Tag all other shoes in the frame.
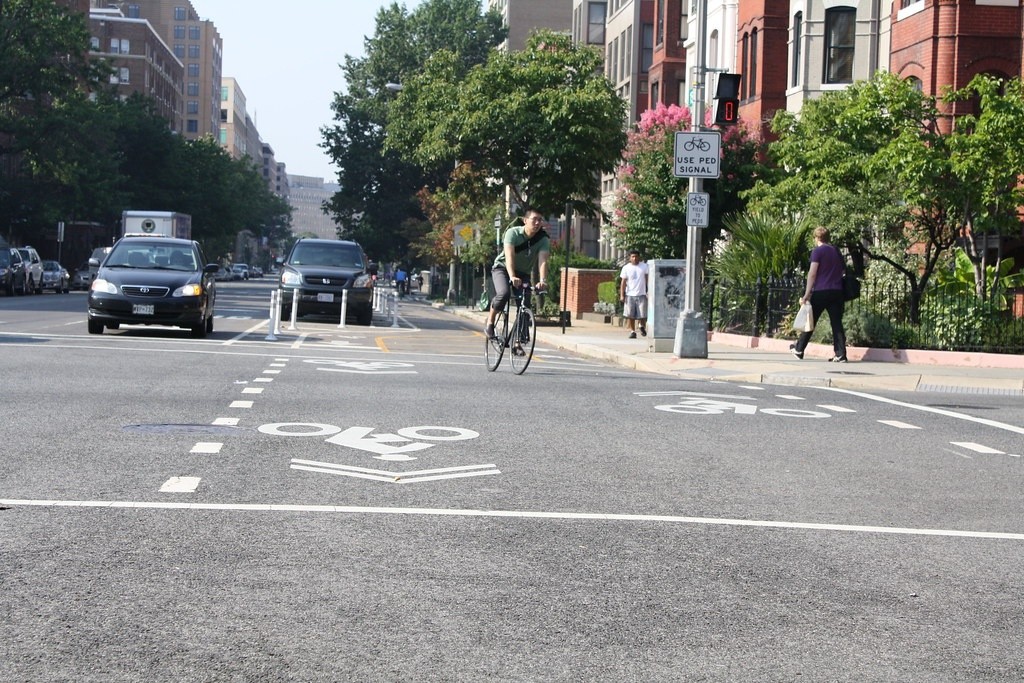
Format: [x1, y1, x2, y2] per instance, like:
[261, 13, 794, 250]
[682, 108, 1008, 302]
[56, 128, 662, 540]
[629, 332, 637, 339]
[512, 346, 526, 356]
[640, 327, 647, 337]
[484, 318, 495, 338]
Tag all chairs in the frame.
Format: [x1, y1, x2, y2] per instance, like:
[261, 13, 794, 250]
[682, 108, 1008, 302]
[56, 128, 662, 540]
[302, 249, 356, 269]
[155, 256, 169, 263]
[129, 252, 144, 266]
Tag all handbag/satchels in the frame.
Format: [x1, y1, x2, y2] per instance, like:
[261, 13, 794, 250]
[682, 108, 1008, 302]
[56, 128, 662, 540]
[501, 217, 549, 253]
[841, 274, 862, 301]
[792, 298, 814, 332]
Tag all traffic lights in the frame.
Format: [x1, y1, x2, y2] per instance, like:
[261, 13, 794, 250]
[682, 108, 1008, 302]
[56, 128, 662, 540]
[714, 72, 742, 125]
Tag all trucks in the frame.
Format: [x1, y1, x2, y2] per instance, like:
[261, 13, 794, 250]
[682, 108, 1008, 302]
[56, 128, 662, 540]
[118, 209, 192, 268]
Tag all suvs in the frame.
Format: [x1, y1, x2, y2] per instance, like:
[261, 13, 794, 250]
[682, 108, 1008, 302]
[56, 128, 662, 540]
[14, 245, 44, 296]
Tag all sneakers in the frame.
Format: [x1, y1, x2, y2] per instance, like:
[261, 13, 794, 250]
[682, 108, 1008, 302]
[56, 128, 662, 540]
[790, 344, 804, 360]
[829, 355, 847, 363]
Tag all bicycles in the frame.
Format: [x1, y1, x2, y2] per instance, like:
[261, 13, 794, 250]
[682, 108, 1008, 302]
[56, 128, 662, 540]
[483, 278, 548, 376]
[395, 280, 406, 301]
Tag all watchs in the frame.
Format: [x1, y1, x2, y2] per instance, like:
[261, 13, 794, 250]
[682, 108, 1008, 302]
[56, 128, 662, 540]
[539, 278, 547, 283]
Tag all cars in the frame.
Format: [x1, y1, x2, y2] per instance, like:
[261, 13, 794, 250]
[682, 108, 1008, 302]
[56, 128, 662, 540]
[0, 242, 28, 298]
[71, 261, 90, 291]
[41, 259, 70, 294]
[273, 235, 376, 327]
[86, 232, 220, 338]
[210, 262, 264, 282]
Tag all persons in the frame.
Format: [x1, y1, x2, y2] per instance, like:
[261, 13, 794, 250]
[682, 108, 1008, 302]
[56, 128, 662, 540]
[397, 269, 407, 297]
[417, 273, 423, 293]
[620, 251, 649, 338]
[483, 208, 549, 357]
[789, 226, 848, 362]
